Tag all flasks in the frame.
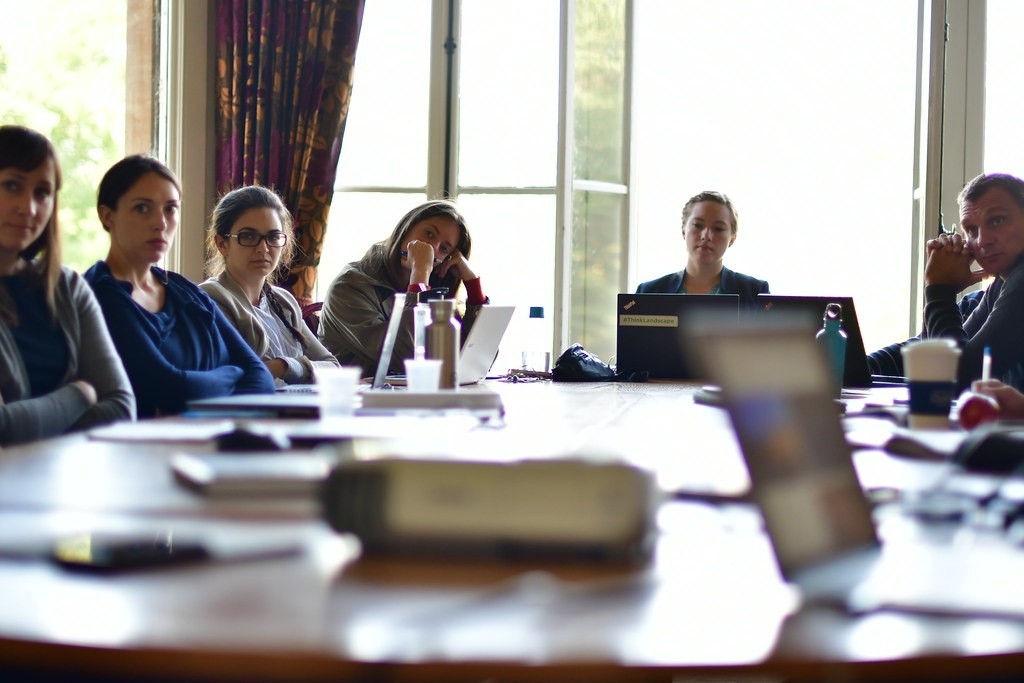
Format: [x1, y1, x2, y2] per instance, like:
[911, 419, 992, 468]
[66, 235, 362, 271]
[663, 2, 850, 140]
[423, 297, 457, 392]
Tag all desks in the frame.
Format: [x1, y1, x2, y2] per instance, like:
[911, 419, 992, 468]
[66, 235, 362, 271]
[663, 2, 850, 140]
[0, 381, 1024, 683]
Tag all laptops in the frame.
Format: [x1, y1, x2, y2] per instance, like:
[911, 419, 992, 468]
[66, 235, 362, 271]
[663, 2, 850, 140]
[618, 294, 1024, 625]
[364, 305, 515, 385]
[273, 294, 406, 396]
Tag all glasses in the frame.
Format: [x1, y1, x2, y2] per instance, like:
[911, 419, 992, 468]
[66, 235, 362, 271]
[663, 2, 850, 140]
[223, 231, 288, 248]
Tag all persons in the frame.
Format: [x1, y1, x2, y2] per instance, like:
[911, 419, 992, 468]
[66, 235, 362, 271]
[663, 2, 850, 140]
[195, 186, 340, 385]
[867, 173, 1024, 423]
[76, 154, 275, 419]
[636, 191, 771, 295]
[0, 124, 136, 447]
[319, 200, 489, 375]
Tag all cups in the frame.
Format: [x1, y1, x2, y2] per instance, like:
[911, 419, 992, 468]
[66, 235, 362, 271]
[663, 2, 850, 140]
[403, 360, 444, 392]
[311, 366, 364, 420]
[899, 336, 962, 432]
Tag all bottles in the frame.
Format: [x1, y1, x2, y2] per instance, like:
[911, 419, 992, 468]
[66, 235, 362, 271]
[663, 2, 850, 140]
[815, 303, 847, 396]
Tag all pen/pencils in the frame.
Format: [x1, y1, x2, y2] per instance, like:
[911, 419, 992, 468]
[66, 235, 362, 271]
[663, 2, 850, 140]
[982, 347, 991, 380]
[401, 251, 443, 262]
